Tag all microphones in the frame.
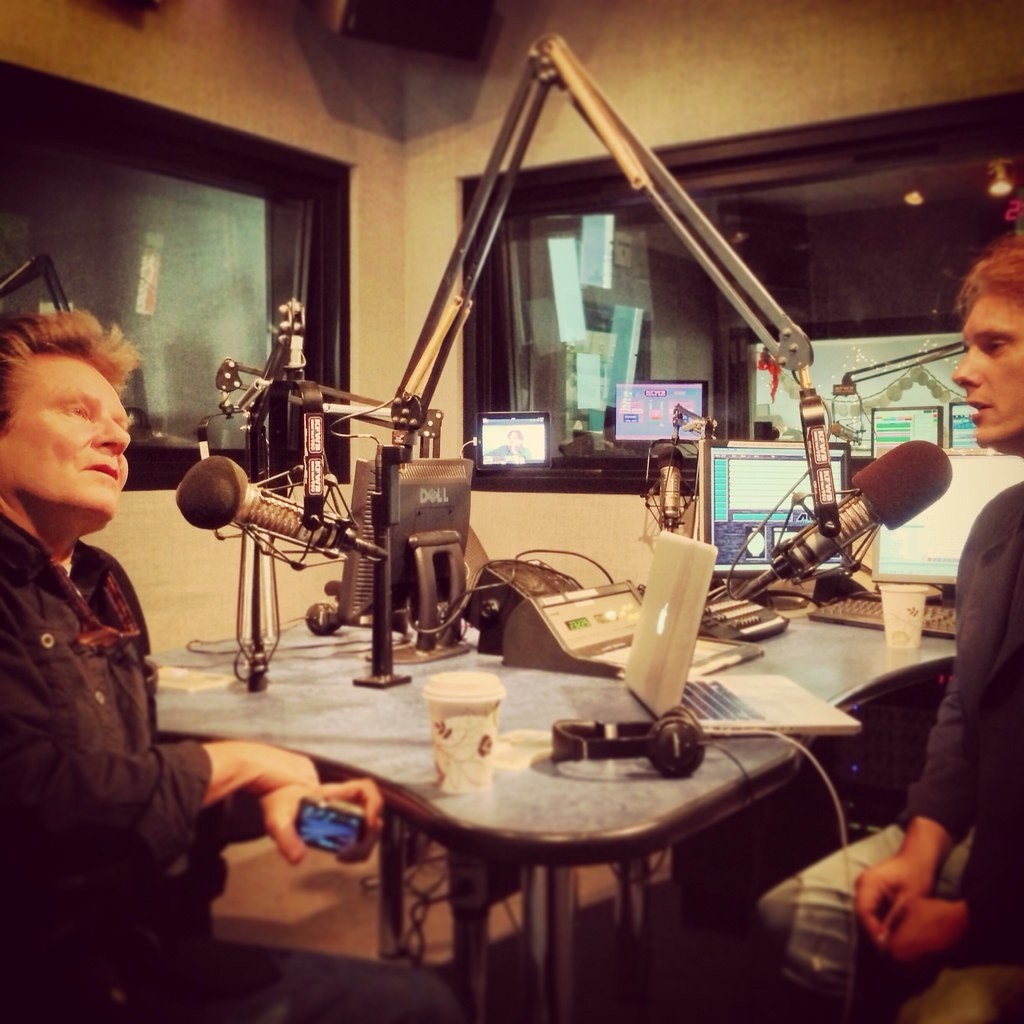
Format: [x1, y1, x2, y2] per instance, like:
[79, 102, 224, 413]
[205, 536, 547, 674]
[175, 455, 389, 560]
[736, 441, 953, 601]
[655, 445, 685, 529]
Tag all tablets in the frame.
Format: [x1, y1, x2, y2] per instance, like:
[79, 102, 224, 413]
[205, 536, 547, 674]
[476, 411, 552, 470]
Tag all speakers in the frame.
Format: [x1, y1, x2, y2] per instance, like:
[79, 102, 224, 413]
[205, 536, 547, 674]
[320, 0, 494, 62]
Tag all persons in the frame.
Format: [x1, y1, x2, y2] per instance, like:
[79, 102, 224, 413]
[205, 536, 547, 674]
[496, 433, 533, 465]
[0, 309, 386, 1024]
[755, 233, 1024, 1024]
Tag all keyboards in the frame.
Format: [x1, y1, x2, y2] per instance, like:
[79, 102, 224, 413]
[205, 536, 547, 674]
[807, 599, 959, 640]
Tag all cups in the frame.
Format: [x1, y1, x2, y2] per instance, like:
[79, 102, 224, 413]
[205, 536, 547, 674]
[423, 670, 506, 795]
[879, 584, 929, 650]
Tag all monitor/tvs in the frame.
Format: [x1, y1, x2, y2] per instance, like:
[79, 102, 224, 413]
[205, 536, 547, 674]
[611, 380, 709, 446]
[949, 401, 982, 450]
[336, 456, 475, 662]
[871, 405, 942, 459]
[872, 448, 1024, 607]
[698, 440, 853, 609]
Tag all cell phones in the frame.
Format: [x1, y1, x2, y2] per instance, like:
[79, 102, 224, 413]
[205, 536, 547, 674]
[297, 793, 366, 854]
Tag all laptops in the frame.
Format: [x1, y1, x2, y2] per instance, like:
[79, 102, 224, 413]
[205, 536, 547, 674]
[626, 532, 863, 736]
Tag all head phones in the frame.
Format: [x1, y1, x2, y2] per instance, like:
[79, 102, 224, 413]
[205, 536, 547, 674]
[550, 704, 707, 777]
[304, 602, 377, 635]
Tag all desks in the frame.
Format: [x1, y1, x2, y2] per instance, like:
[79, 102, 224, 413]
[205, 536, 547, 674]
[150, 599, 958, 1024]
